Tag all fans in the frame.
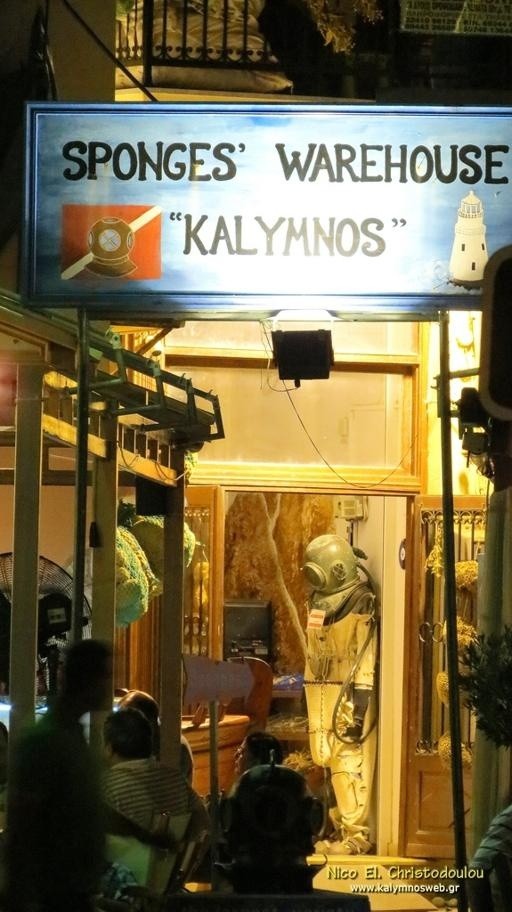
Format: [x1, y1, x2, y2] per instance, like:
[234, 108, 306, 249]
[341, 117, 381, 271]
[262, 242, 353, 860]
[0, 552, 92, 709]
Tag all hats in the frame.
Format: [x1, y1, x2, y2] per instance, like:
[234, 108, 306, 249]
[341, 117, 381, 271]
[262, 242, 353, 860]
[39, 595, 88, 640]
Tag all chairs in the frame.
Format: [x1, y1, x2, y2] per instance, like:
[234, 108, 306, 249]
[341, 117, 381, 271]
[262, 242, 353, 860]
[146, 789, 226, 896]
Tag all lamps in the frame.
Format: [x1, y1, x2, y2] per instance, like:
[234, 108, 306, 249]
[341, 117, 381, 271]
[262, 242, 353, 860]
[271, 328, 334, 387]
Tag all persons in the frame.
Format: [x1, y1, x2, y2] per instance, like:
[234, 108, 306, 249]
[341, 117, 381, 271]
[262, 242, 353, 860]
[301, 532, 379, 854]
[1, 638, 284, 912]
[467, 805, 512, 912]
